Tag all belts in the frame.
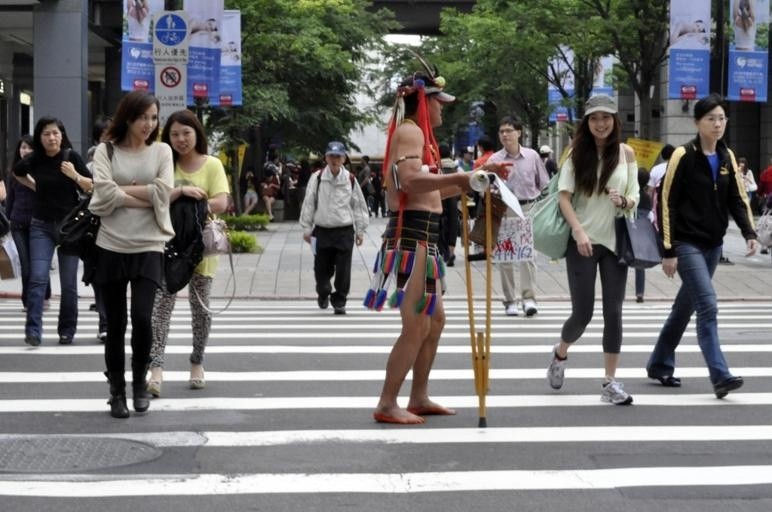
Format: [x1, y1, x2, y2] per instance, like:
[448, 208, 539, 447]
[519, 200, 534, 204]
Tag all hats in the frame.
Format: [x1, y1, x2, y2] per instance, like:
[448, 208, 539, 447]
[425, 87, 456, 102]
[325, 142, 346, 156]
[584, 94, 618, 116]
[540, 145, 554, 154]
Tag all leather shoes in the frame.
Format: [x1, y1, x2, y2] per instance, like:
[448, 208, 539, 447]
[189, 367, 206, 388]
[713, 376, 743, 399]
[147, 379, 161, 397]
[648, 375, 680, 387]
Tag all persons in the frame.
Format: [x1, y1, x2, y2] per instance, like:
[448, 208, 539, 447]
[758, 167, 772, 254]
[241, 171, 263, 216]
[733, 0, 757, 51]
[264, 152, 326, 215]
[190, 18, 217, 33]
[364, 76, 515, 423]
[299, 141, 369, 314]
[540, 145, 558, 179]
[720, 247, 733, 265]
[485, 115, 550, 317]
[261, 169, 280, 221]
[678, 20, 706, 37]
[438, 135, 495, 267]
[127, 0, 151, 45]
[89, 90, 174, 419]
[635, 168, 655, 302]
[737, 158, 755, 200]
[1, 115, 111, 346]
[548, 94, 640, 405]
[353, 156, 388, 218]
[648, 144, 675, 197]
[147, 111, 231, 399]
[645, 97, 758, 398]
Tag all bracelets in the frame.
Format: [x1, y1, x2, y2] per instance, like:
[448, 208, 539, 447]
[180, 185, 183, 195]
[75, 174, 79, 181]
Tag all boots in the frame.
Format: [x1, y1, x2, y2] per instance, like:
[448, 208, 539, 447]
[105, 371, 129, 418]
[131, 355, 153, 412]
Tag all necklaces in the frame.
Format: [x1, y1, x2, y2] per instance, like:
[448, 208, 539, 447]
[403, 120, 435, 155]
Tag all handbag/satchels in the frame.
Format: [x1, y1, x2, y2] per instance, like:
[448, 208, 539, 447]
[755, 216, 771, 248]
[202, 214, 228, 257]
[528, 173, 576, 260]
[615, 215, 661, 270]
[58, 198, 101, 263]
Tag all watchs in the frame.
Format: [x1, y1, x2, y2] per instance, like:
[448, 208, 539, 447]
[620, 196, 627, 209]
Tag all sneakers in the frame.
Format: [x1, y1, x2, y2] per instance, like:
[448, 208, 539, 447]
[600, 382, 632, 404]
[58, 335, 72, 344]
[97, 332, 107, 342]
[25, 333, 39, 346]
[506, 302, 518, 315]
[318, 296, 328, 308]
[334, 305, 345, 315]
[523, 298, 536, 316]
[547, 344, 567, 389]
